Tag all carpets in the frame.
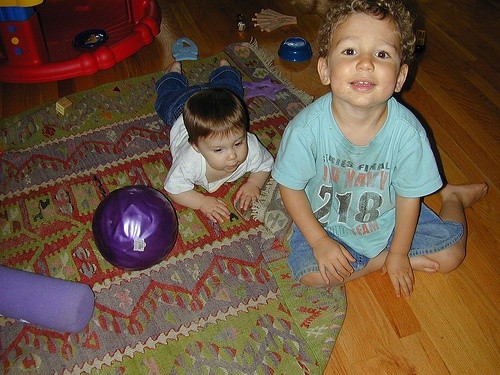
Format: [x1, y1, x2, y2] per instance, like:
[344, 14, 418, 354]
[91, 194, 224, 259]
[0, 37, 348, 375]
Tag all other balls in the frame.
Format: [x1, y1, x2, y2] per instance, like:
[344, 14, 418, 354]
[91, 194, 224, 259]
[91, 185, 179, 270]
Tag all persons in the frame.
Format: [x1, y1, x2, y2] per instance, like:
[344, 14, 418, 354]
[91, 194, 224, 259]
[271, 0, 488, 300]
[154, 58, 275, 223]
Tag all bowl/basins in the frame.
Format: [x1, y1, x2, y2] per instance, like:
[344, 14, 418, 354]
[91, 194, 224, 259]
[278, 37, 313, 62]
